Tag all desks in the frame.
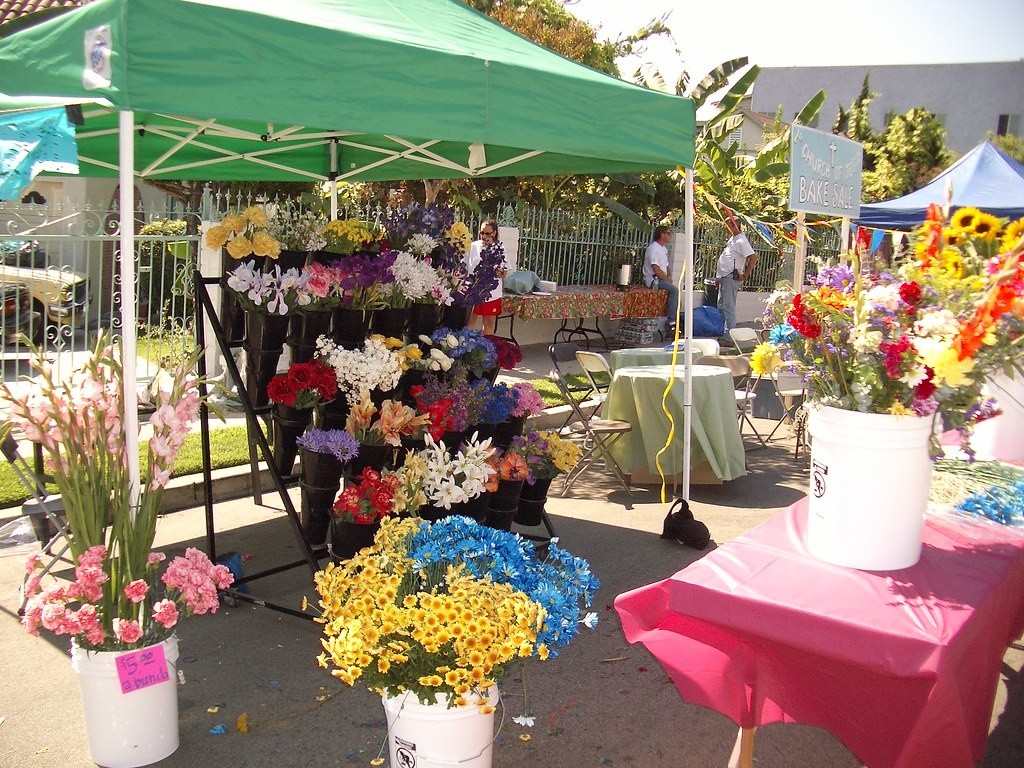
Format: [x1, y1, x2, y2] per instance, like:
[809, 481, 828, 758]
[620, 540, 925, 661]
[495, 284, 667, 351]
[597, 365, 748, 486]
[609, 347, 704, 370]
[615, 492, 1024, 768]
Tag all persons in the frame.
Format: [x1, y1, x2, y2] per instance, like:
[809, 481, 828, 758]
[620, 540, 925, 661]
[461, 219, 512, 335]
[716, 216, 756, 347]
[643, 225, 683, 340]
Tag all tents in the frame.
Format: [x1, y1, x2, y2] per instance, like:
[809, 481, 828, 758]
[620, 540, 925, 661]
[0, 0, 695, 530]
[851, 141, 1024, 235]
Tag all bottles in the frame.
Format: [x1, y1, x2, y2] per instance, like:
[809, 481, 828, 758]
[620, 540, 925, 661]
[653, 277, 658, 290]
[614, 317, 658, 345]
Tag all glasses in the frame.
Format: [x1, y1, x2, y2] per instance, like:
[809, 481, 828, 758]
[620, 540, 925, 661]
[664, 232, 671, 235]
[480, 230, 495, 236]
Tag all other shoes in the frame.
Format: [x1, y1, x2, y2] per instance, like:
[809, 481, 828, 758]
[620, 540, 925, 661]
[717, 337, 734, 347]
[666, 322, 681, 335]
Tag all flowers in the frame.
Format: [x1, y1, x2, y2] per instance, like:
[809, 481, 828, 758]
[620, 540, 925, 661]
[0, 327, 236, 652]
[205, 202, 600, 768]
[754, 197, 1024, 459]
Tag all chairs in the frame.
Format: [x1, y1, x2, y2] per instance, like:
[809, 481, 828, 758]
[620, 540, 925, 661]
[548, 343, 609, 437]
[729, 328, 773, 409]
[695, 354, 768, 452]
[574, 351, 616, 452]
[550, 370, 636, 498]
[766, 364, 808, 452]
[21, 435, 66, 554]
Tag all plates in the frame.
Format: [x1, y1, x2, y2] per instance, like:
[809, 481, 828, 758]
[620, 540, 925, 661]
[532, 292, 552, 296]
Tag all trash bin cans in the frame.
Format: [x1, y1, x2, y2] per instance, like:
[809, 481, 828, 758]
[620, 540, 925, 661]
[750, 370, 786, 420]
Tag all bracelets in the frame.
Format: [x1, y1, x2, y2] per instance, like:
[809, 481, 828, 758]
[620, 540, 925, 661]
[741, 273, 748, 280]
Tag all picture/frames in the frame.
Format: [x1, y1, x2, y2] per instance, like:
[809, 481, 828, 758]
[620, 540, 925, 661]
[381, 682, 500, 768]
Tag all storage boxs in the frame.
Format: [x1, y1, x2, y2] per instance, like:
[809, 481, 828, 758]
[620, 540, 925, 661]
[615, 314, 660, 345]
[749, 372, 805, 418]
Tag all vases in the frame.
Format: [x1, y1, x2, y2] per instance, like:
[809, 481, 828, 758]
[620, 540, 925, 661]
[408, 303, 441, 359]
[456, 491, 492, 526]
[370, 307, 409, 342]
[395, 439, 425, 468]
[419, 499, 457, 524]
[332, 518, 382, 567]
[805, 400, 940, 570]
[440, 302, 474, 332]
[515, 478, 551, 526]
[298, 447, 343, 547]
[488, 478, 525, 534]
[369, 387, 388, 426]
[332, 309, 372, 351]
[402, 370, 434, 409]
[287, 307, 333, 364]
[440, 431, 467, 457]
[240, 304, 291, 406]
[265, 249, 309, 278]
[344, 444, 394, 491]
[312, 252, 347, 266]
[316, 386, 351, 431]
[491, 415, 529, 454]
[467, 423, 495, 445]
[272, 402, 313, 476]
[468, 364, 501, 390]
[69, 636, 182, 768]
[221, 245, 268, 345]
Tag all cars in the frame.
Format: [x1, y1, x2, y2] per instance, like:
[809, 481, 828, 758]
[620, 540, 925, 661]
[0, 280, 41, 347]
[0, 264, 92, 345]
